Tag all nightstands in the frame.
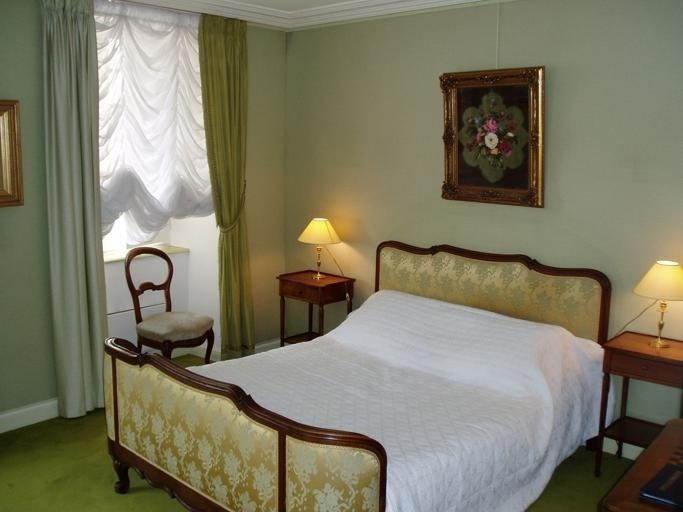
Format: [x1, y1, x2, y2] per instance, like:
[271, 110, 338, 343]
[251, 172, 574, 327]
[275, 269, 357, 347]
[594, 329, 683, 478]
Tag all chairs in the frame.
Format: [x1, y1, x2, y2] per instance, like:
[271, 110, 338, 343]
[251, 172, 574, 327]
[124, 246, 214, 364]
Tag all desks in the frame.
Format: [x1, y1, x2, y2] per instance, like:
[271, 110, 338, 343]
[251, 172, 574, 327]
[600, 418, 683, 512]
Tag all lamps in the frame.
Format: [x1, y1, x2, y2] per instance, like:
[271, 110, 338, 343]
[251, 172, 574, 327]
[297, 217, 342, 280]
[632, 258, 683, 348]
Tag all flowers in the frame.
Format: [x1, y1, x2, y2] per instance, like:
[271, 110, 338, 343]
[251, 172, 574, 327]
[458, 92, 527, 184]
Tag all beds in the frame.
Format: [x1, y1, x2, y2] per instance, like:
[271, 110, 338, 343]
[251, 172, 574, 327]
[101, 239, 616, 512]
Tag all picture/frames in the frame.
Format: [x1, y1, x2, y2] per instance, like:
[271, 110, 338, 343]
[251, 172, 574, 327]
[438, 64, 545, 208]
[0, 98, 24, 206]
